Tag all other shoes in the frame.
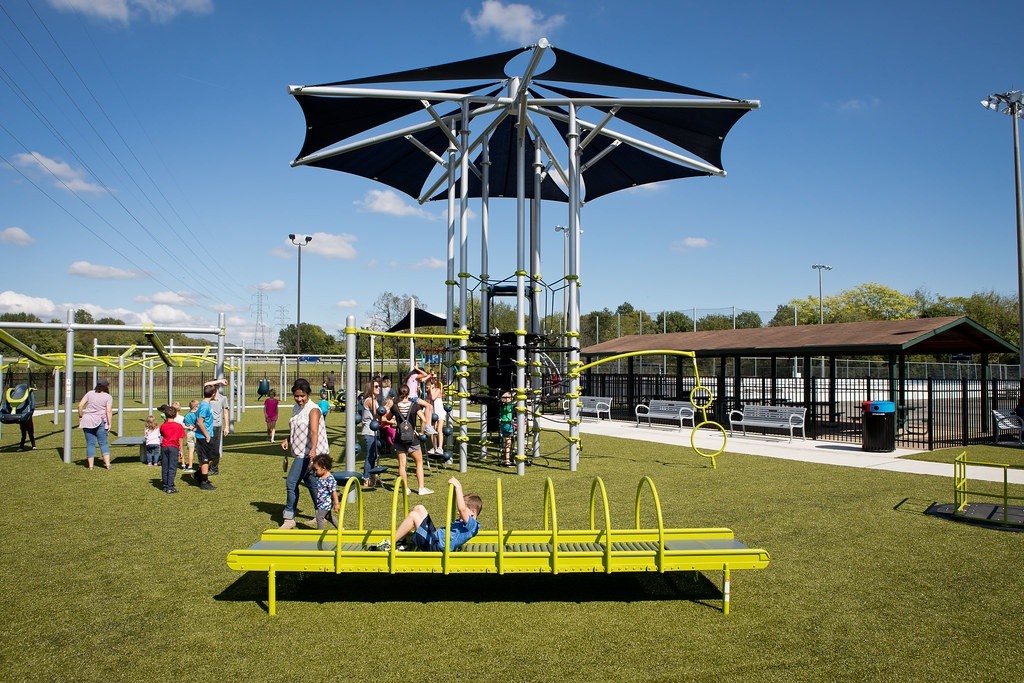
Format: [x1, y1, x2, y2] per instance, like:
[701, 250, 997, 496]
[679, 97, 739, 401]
[167, 489, 176, 493]
[148, 462, 152, 466]
[32, 447, 37, 450]
[369, 538, 393, 551]
[201, 481, 216, 489]
[187, 467, 193, 472]
[153, 463, 159, 466]
[406, 487, 411, 495]
[163, 486, 168, 491]
[425, 425, 438, 434]
[428, 448, 435, 454]
[181, 465, 186, 471]
[17, 448, 23, 452]
[270, 439, 275, 444]
[396, 538, 408, 551]
[89, 467, 94, 470]
[278, 519, 297, 530]
[419, 487, 433, 495]
[105, 464, 111, 469]
[435, 448, 443, 455]
[363, 479, 377, 486]
[211, 466, 218, 475]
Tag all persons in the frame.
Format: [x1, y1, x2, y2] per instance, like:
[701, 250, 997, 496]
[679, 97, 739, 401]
[322, 380, 327, 390]
[182, 400, 199, 472]
[499, 392, 517, 467]
[318, 393, 329, 420]
[387, 385, 435, 496]
[402, 364, 438, 435]
[16, 391, 36, 452]
[144, 416, 161, 465]
[381, 409, 396, 446]
[370, 477, 483, 551]
[380, 376, 399, 454]
[327, 370, 339, 400]
[312, 454, 345, 529]
[195, 385, 219, 491]
[361, 380, 391, 487]
[5, 384, 37, 414]
[204, 379, 230, 476]
[277, 379, 329, 530]
[160, 406, 186, 493]
[172, 400, 186, 470]
[263, 389, 279, 443]
[418, 378, 446, 455]
[77, 377, 113, 470]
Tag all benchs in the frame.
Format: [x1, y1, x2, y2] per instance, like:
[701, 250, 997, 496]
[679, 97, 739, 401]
[728, 405, 809, 445]
[991, 406, 1023, 450]
[562, 394, 612, 423]
[633, 399, 696, 430]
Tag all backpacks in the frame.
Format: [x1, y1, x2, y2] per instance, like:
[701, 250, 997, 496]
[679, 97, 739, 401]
[394, 402, 419, 443]
[255, 372, 271, 401]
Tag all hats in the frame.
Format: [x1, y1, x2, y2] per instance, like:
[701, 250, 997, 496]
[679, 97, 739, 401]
[97, 379, 108, 387]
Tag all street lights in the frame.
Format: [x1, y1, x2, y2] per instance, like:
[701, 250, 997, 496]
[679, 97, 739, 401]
[980, 89, 1022, 400]
[555, 224, 583, 374]
[288, 233, 311, 384]
[813, 263, 832, 324]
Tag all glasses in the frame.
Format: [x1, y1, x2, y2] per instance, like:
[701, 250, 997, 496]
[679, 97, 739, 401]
[426, 386, 429, 389]
[371, 385, 381, 389]
